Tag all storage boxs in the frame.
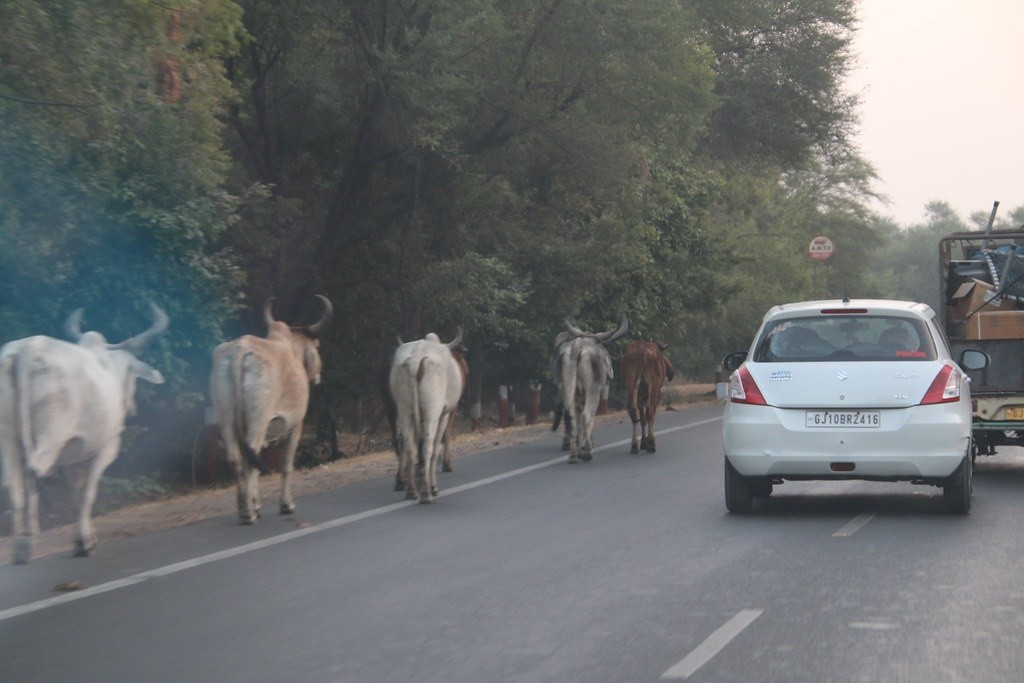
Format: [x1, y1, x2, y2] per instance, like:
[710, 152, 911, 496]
[965, 310, 1024, 340]
[951, 278, 1017, 319]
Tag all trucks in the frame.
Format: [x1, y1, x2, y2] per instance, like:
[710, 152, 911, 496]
[934, 226, 1024, 463]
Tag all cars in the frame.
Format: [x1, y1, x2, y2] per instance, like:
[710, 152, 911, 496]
[716, 297, 992, 518]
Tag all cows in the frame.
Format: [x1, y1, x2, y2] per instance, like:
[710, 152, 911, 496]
[202, 290, 336, 525]
[0, 299, 171, 566]
[549, 312, 676, 465]
[383, 322, 469, 506]
